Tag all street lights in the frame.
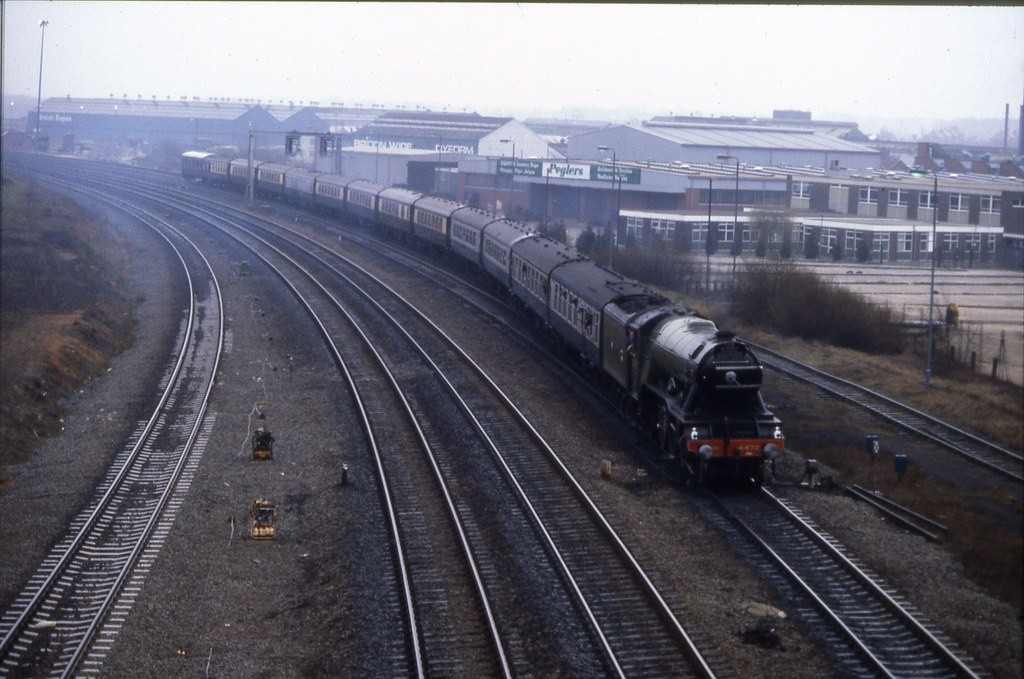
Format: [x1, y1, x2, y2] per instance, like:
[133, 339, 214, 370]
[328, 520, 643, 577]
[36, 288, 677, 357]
[431, 132, 443, 199]
[500, 140, 517, 221]
[34, 20, 51, 133]
[597, 146, 617, 271]
[909, 168, 939, 388]
[717, 153, 739, 287]
[686, 176, 713, 297]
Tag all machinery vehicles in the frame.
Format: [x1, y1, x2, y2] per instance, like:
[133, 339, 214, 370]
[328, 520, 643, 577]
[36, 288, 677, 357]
[249, 413, 277, 460]
[248, 497, 279, 541]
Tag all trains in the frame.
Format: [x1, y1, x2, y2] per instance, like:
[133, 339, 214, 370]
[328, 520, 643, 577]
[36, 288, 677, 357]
[177, 149, 789, 495]
